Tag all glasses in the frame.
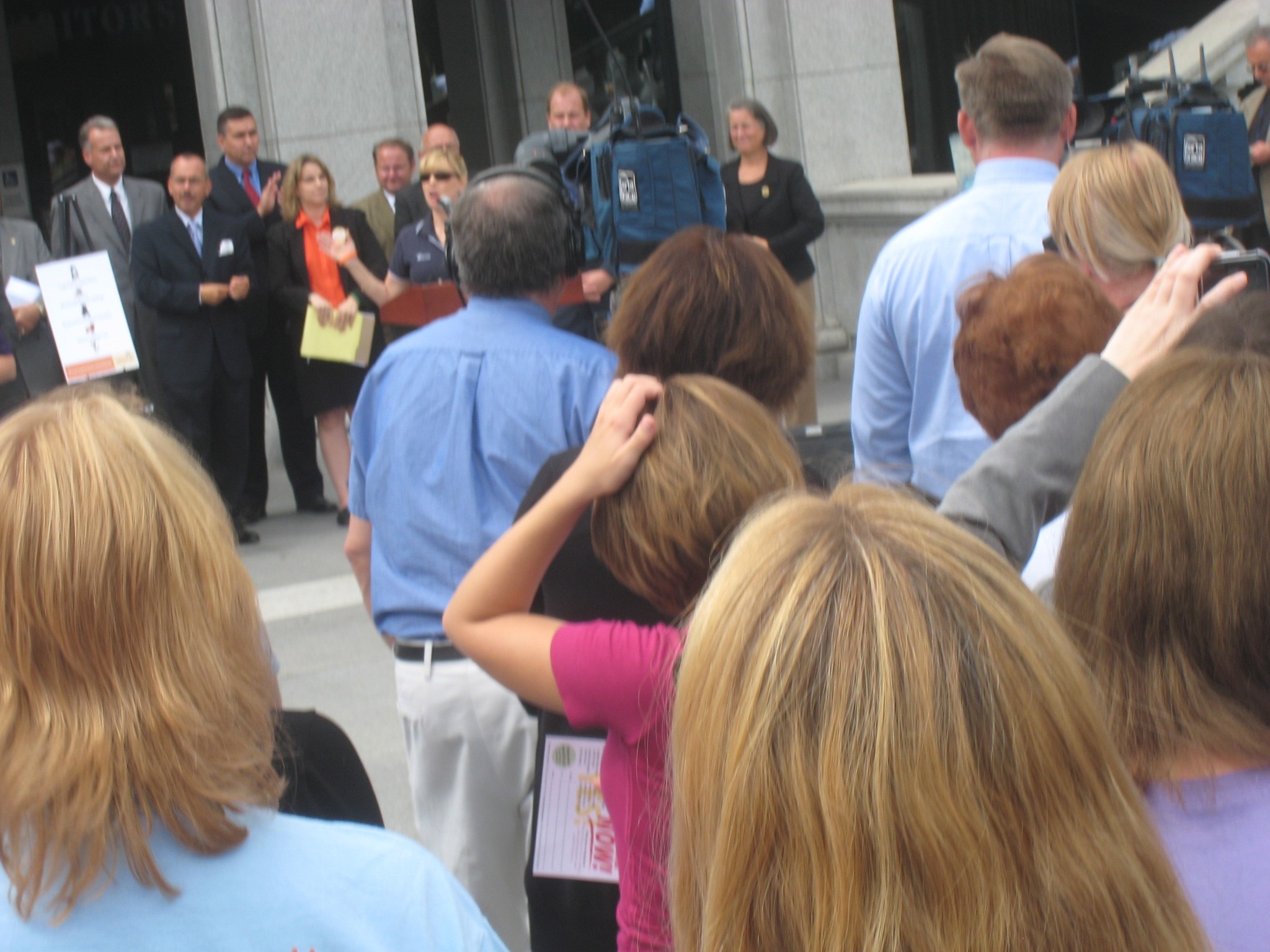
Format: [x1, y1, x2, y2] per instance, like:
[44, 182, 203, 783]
[421, 172, 452, 181]
[1043, 235, 1061, 252]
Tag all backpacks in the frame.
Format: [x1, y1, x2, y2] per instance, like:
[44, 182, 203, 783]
[561, 103, 727, 331]
[1115, 78, 1260, 241]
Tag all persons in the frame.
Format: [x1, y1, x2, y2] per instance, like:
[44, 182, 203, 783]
[124, 147, 261, 546]
[345, 167, 647, 951]
[0, 30, 1269, 952]
[851, 32, 1082, 504]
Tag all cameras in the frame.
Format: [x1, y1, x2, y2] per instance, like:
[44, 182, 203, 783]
[1196, 247, 1270, 308]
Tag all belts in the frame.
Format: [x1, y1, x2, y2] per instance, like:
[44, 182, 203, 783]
[394, 645, 465, 662]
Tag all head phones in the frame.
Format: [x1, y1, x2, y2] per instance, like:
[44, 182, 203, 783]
[442, 163, 587, 277]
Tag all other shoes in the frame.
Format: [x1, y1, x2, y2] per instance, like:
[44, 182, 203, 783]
[234, 525, 259, 544]
[300, 500, 328, 515]
[337, 508, 350, 525]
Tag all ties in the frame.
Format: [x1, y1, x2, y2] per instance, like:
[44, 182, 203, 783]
[190, 221, 203, 257]
[110, 191, 131, 255]
[242, 170, 261, 210]
